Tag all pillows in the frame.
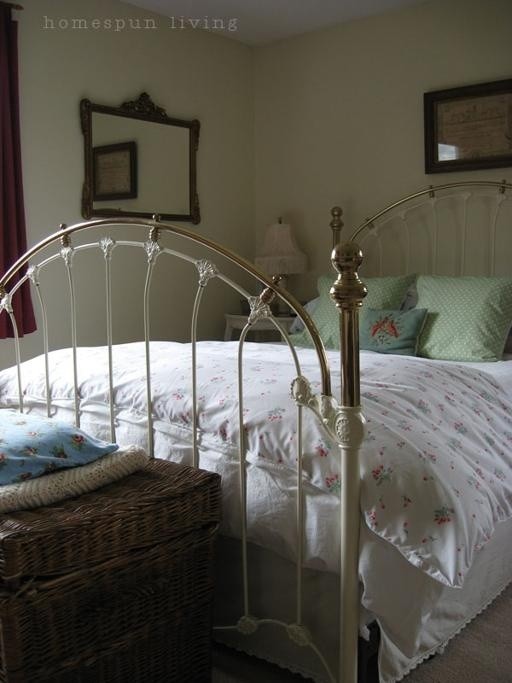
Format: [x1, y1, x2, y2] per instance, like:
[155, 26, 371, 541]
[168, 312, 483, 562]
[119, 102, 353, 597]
[281, 274, 512, 363]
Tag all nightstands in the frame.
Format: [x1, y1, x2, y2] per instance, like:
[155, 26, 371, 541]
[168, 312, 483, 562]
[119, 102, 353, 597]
[224, 310, 299, 343]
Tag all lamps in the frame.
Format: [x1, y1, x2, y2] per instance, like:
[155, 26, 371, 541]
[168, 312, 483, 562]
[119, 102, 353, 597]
[252, 219, 308, 316]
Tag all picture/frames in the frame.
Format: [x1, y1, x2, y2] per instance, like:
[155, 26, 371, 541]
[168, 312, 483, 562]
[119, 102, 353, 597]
[422, 78, 512, 174]
[92, 139, 137, 204]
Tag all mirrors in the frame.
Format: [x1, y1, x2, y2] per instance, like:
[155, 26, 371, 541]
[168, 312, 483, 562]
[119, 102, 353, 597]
[79, 90, 202, 221]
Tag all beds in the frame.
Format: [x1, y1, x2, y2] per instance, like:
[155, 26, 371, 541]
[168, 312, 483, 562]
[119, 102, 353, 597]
[0, 180, 512, 682]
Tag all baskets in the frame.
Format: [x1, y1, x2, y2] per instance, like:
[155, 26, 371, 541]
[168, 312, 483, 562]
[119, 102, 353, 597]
[2, 457, 225, 683]
